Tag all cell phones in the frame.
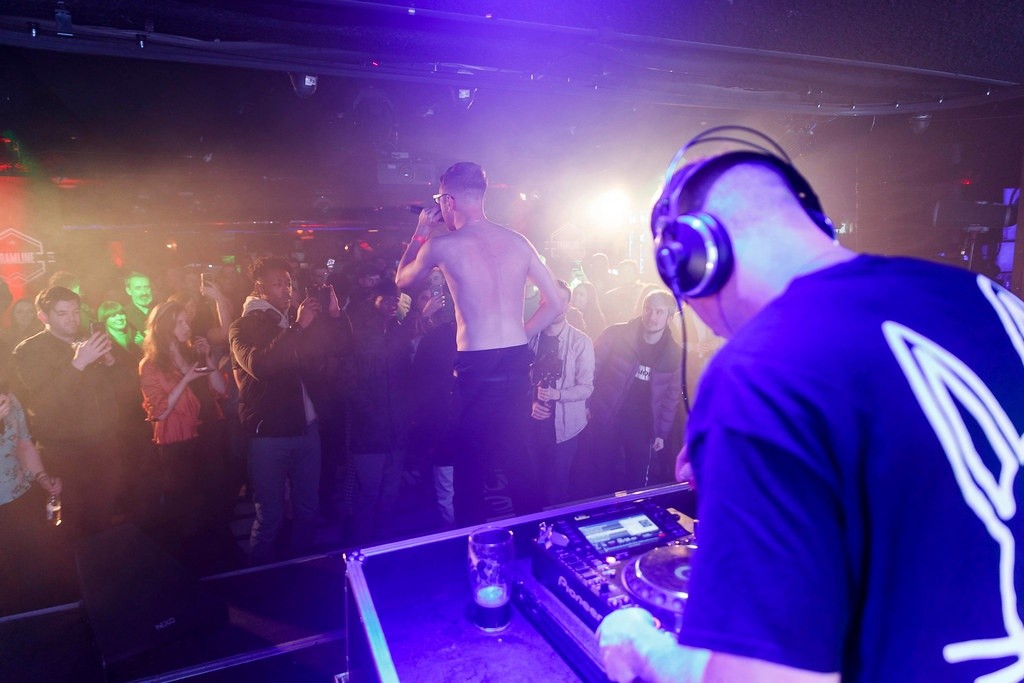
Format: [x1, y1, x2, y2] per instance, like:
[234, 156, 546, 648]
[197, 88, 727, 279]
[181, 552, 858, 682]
[201, 272, 213, 295]
[432, 284, 443, 297]
[399, 293, 411, 310]
[305, 285, 330, 313]
[92, 322, 106, 344]
[195, 368, 216, 374]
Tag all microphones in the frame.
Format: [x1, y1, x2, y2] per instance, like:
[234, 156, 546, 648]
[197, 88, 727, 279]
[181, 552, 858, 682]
[406, 204, 444, 222]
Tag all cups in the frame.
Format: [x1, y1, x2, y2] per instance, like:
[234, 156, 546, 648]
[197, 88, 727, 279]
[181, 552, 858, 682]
[468, 525, 514, 634]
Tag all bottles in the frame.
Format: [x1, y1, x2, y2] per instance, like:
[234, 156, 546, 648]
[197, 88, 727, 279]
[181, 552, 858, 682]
[45, 494, 62, 527]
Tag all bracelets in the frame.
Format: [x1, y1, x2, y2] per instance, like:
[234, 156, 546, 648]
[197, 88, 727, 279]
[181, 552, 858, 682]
[411, 233, 425, 245]
[35, 472, 49, 484]
[291, 322, 304, 334]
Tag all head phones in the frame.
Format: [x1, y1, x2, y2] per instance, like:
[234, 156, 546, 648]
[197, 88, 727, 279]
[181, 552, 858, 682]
[655, 124, 838, 298]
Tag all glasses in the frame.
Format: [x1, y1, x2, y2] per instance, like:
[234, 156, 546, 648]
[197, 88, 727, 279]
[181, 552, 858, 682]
[433, 193, 454, 205]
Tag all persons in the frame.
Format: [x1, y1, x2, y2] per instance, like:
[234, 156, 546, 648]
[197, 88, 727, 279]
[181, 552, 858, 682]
[594, 126, 1024, 683]
[0, 188, 1024, 615]
[393, 161, 565, 528]
